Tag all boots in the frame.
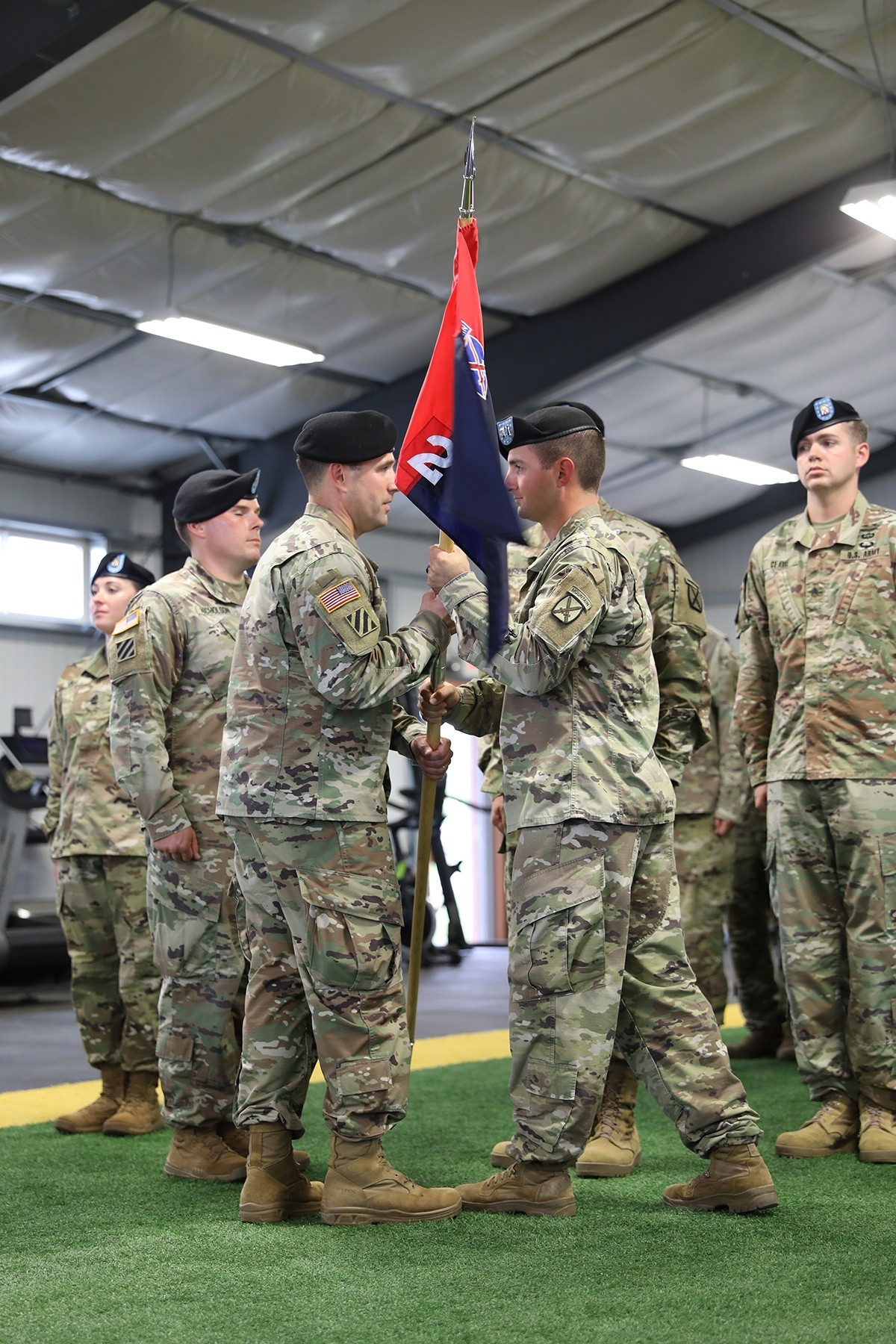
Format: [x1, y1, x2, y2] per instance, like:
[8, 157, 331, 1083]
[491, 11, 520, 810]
[573, 1064, 644, 1178]
[239, 1124, 325, 1224]
[102, 1070, 164, 1135]
[318, 1133, 463, 1226]
[53, 1068, 130, 1135]
[488, 1139, 519, 1171]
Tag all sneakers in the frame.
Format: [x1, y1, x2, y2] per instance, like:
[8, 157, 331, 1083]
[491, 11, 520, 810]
[216, 1123, 310, 1171]
[773, 1090, 861, 1159]
[163, 1124, 251, 1184]
[659, 1142, 780, 1214]
[453, 1159, 579, 1219]
[858, 1097, 896, 1163]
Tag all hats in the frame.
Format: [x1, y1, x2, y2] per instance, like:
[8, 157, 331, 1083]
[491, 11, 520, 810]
[790, 396, 863, 461]
[169, 468, 265, 524]
[88, 550, 156, 586]
[293, 408, 400, 465]
[496, 401, 605, 462]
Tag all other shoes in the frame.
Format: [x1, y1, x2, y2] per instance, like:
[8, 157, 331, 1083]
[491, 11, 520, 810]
[725, 1028, 778, 1059]
[773, 1037, 799, 1058]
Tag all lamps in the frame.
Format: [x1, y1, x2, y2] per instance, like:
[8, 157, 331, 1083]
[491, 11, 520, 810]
[681, 382, 799, 486]
[838, 180, 895, 240]
[133, 222, 325, 368]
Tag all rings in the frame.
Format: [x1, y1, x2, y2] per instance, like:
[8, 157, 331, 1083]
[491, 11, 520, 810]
[425, 565, 431, 573]
[443, 766, 447, 771]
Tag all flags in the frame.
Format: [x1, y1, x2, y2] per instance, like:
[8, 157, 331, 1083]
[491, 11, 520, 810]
[393, 210, 537, 663]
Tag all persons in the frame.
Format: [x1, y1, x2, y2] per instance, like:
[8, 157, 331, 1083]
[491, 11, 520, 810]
[732, 396, 896, 1163]
[488, 399, 806, 1180]
[44, 552, 159, 1135]
[108, 462, 314, 1185]
[216, 409, 464, 1226]
[418, 405, 788, 1217]
